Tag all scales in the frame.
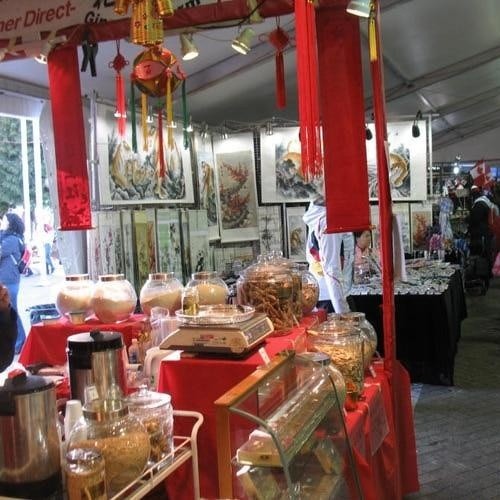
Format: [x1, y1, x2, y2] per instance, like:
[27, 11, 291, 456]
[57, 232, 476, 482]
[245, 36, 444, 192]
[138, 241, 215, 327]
[160, 304, 275, 360]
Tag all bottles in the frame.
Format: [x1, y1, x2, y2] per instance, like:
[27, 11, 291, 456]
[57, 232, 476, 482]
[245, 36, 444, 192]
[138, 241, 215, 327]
[90, 273, 138, 325]
[122, 382, 177, 471]
[56, 272, 94, 320]
[184, 271, 230, 306]
[306, 311, 379, 416]
[128, 338, 139, 365]
[237, 250, 320, 337]
[139, 272, 184, 317]
[64, 397, 152, 500]
[180, 286, 201, 316]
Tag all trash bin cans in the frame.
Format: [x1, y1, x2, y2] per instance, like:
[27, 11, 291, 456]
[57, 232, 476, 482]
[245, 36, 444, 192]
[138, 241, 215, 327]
[27, 305, 58, 324]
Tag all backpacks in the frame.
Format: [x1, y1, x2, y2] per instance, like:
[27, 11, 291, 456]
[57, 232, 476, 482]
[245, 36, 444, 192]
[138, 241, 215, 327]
[471, 196, 500, 235]
[2, 235, 33, 275]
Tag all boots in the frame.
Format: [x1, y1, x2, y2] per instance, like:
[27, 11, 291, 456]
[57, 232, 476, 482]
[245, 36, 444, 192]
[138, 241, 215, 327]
[46, 263, 56, 275]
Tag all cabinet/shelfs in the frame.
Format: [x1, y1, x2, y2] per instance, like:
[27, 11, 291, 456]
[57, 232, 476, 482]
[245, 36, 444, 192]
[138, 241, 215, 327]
[107, 410, 204, 500]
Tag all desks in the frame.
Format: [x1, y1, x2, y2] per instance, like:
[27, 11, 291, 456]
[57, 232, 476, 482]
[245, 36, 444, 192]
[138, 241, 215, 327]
[344, 261, 460, 384]
[18, 309, 153, 367]
[158, 308, 420, 499]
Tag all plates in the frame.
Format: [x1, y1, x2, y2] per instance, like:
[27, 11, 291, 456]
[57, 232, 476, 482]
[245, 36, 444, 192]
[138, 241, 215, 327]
[173, 304, 256, 325]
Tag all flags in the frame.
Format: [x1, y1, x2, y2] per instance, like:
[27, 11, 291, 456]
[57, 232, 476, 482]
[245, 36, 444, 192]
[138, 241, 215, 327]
[478, 159, 496, 187]
[471, 159, 487, 189]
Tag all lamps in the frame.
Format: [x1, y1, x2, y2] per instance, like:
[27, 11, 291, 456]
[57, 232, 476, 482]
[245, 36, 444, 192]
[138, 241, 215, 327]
[231, 24, 260, 57]
[411, 110, 422, 138]
[346, 1, 373, 19]
[365, 113, 374, 138]
[114, 101, 275, 139]
[178, 33, 200, 61]
[1, 30, 70, 64]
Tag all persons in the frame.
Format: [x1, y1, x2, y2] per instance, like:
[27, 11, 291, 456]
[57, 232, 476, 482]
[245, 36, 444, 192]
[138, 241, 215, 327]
[43, 223, 56, 275]
[1, 284, 19, 374]
[465, 184, 499, 285]
[354, 229, 373, 272]
[0, 212, 33, 355]
[304, 178, 357, 312]
